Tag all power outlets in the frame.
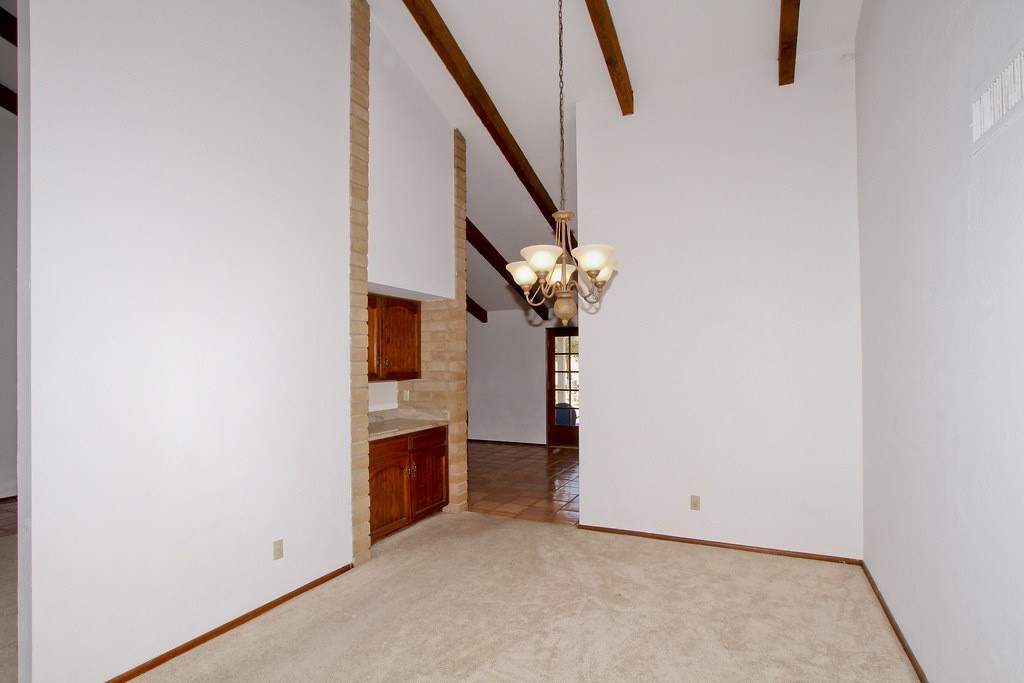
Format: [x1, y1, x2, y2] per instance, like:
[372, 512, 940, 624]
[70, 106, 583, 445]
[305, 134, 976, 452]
[403, 389, 409, 402]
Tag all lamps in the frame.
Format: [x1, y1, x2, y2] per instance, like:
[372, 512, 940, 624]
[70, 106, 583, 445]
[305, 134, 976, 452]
[506, 0, 617, 326]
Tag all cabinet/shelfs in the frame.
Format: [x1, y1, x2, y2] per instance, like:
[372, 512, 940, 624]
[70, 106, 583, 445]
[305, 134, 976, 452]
[368, 291, 422, 381]
[369, 425, 450, 544]
